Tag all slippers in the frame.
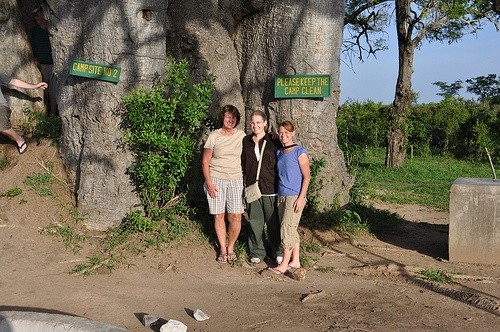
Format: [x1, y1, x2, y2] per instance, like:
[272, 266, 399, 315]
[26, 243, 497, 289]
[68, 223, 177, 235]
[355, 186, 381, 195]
[271, 264, 301, 275]
[18, 138, 28, 154]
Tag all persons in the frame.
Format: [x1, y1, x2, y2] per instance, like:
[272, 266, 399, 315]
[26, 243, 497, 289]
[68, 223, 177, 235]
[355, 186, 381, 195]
[203, 104, 248, 264]
[0, 75, 48, 154]
[241, 110, 286, 264]
[269, 120, 311, 275]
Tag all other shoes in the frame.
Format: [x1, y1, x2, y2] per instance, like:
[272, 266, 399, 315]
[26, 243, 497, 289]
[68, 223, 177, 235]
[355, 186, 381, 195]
[250, 257, 261, 264]
[276, 256, 283, 266]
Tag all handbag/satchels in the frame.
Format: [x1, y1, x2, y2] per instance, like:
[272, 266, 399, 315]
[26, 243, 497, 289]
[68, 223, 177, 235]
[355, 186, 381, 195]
[244, 181, 262, 204]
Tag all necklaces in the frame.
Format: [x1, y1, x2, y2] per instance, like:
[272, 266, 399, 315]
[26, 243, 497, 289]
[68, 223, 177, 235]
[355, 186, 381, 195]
[284, 144, 296, 150]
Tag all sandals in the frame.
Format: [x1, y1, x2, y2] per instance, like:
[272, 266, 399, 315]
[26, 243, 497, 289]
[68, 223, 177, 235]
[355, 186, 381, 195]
[217, 250, 237, 264]
